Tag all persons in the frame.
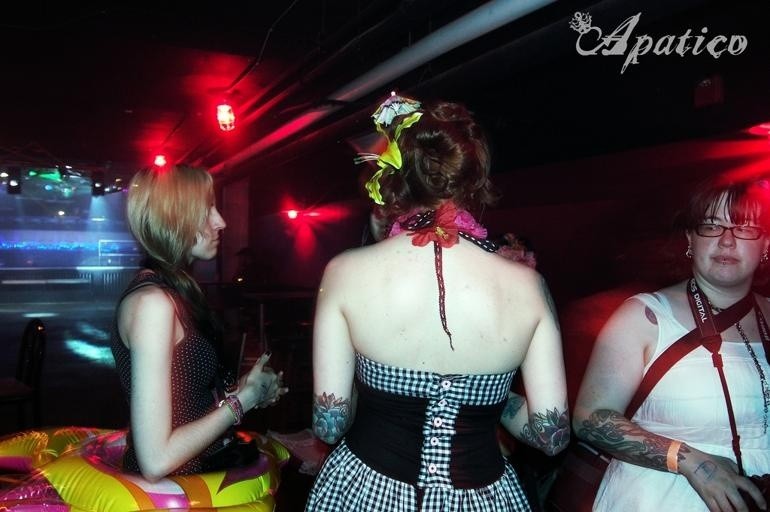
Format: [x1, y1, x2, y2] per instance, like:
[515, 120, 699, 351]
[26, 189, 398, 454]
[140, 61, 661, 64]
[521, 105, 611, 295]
[571, 157, 770, 512]
[304, 95, 571, 512]
[111, 162, 290, 485]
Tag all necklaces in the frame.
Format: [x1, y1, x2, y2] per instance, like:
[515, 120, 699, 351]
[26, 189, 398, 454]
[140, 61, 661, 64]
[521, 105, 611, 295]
[708, 294, 770, 433]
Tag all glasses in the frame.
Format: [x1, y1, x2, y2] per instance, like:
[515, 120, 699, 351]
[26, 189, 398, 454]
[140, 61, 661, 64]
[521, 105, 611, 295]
[695, 223, 762, 240]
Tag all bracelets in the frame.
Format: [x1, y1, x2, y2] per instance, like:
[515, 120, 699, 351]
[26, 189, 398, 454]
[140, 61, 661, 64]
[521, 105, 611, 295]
[666, 438, 681, 474]
[219, 393, 244, 428]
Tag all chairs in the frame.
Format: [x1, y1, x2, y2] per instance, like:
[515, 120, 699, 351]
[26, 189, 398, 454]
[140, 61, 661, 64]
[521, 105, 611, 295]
[0, 318, 48, 436]
